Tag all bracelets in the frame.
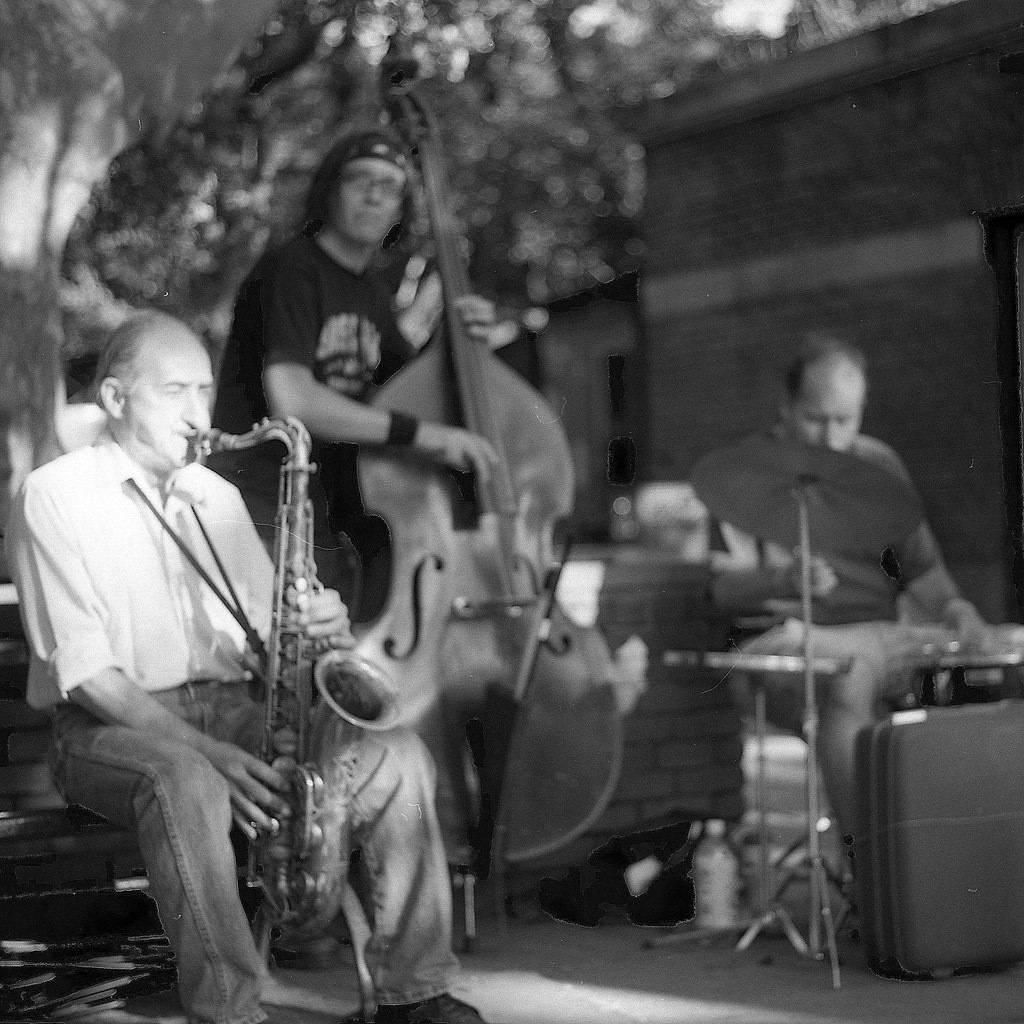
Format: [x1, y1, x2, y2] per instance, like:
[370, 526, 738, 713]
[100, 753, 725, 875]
[385, 409, 419, 449]
[770, 562, 790, 601]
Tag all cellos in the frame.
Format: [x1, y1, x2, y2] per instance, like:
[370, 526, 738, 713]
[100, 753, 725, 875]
[344, 50, 626, 954]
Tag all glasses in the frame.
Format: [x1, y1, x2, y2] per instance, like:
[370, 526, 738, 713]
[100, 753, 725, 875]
[339, 169, 404, 201]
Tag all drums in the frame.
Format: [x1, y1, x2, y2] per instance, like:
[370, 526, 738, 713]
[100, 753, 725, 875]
[909, 644, 1024, 714]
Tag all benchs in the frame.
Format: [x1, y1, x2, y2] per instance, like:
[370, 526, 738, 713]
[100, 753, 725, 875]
[0, 603, 378, 1024]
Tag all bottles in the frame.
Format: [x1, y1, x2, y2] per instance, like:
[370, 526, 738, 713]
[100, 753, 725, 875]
[690, 819, 738, 948]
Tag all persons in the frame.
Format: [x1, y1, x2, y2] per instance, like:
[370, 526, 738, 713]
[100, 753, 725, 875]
[6, 313, 492, 1024]
[207, 126, 500, 591]
[706, 340, 991, 900]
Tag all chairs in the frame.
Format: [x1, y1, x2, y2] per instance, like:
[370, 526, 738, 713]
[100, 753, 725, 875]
[710, 519, 874, 958]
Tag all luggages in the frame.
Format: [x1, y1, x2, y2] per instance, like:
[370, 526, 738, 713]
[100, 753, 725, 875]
[855, 656, 1024, 982]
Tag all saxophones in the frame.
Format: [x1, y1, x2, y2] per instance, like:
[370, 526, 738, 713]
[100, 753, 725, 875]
[181, 410, 404, 946]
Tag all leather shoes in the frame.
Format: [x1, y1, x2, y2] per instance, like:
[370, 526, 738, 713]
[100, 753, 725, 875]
[377, 992, 483, 1024]
